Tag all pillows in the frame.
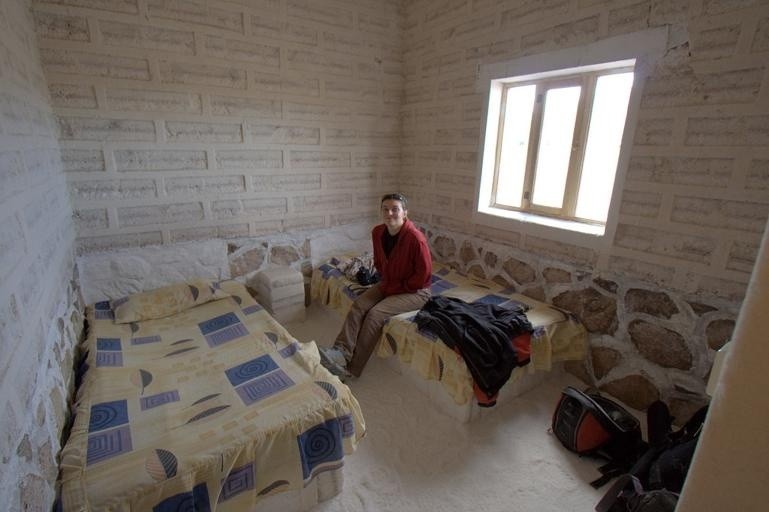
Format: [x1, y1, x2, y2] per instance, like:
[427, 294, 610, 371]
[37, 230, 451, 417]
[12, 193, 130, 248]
[108, 278, 231, 325]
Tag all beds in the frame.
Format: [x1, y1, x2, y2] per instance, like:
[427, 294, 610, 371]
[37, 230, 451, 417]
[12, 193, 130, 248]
[308, 249, 590, 423]
[58, 277, 367, 512]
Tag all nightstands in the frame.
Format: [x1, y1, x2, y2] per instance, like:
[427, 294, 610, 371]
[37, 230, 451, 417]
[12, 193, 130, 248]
[259, 271, 306, 324]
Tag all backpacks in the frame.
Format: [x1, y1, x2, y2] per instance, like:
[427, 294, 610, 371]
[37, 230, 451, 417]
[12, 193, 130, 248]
[594, 473, 680, 512]
[641, 403, 710, 492]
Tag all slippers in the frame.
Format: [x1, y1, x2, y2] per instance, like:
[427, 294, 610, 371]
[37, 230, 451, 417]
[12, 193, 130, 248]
[317, 346, 353, 377]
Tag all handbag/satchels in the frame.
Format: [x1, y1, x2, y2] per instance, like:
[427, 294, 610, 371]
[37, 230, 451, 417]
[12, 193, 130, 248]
[551, 384, 642, 458]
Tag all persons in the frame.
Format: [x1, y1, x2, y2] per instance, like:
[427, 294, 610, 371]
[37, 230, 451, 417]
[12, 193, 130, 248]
[317, 193, 434, 383]
